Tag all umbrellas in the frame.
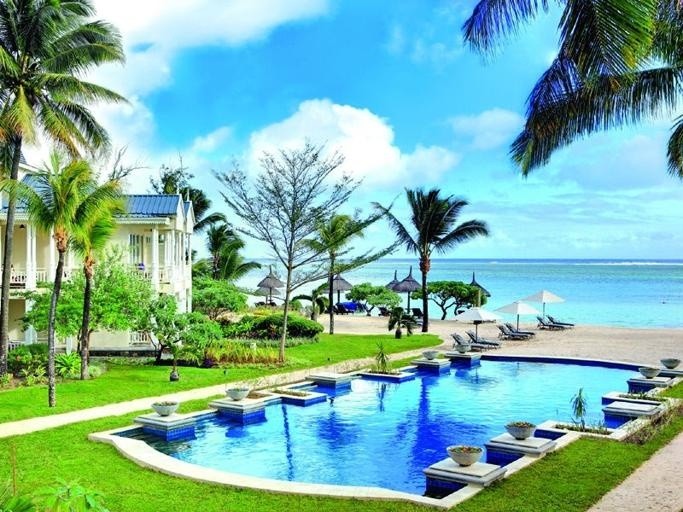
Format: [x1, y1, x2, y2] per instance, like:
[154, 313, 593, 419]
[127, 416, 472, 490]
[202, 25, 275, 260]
[454, 306, 502, 344]
[492, 300, 540, 333]
[522, 289, 565, 317]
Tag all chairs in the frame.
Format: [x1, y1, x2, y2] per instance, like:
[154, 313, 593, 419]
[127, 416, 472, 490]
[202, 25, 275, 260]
[411, 308, 423, 318]
[451, 330, 499, 353]
[497, 322, 535, 340]
[537, 314, 574, 330]
[326, 303, 354, 315]
[378, 307, 391, 317]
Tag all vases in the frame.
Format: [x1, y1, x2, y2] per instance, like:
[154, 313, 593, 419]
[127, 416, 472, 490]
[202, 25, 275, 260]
[152, 401, 177, 416]
[225, 388, 249, 401]
[446, 446, 484, 466]
[639, 368, 661, 378]
[661, 358, 680, 369]
[504, 422, 536, 440]
[424, 351, 438, 360]
